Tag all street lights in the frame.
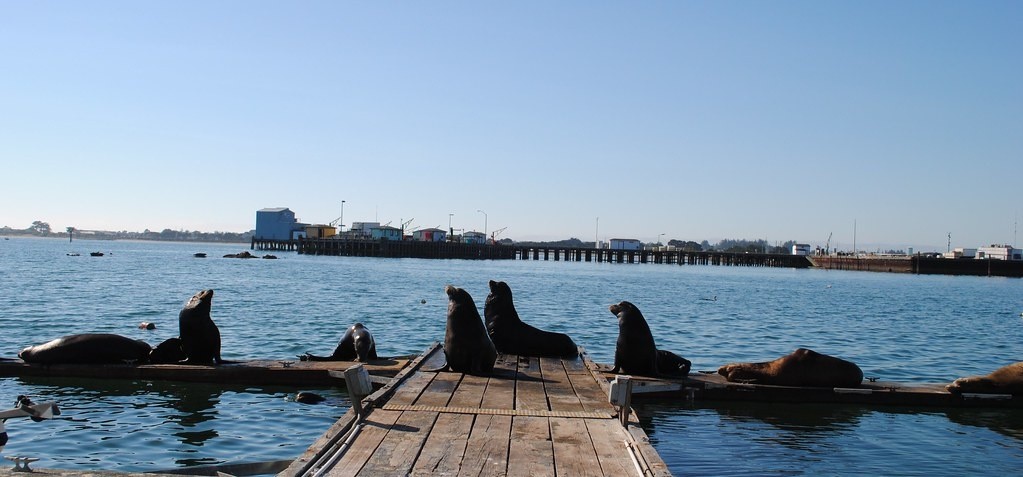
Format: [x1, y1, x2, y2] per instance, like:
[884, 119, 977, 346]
[477, 209, 488, 244]
[340, 200, 346, 231]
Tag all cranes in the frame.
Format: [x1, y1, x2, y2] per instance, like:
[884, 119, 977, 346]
[330, 214, 508, 239]
